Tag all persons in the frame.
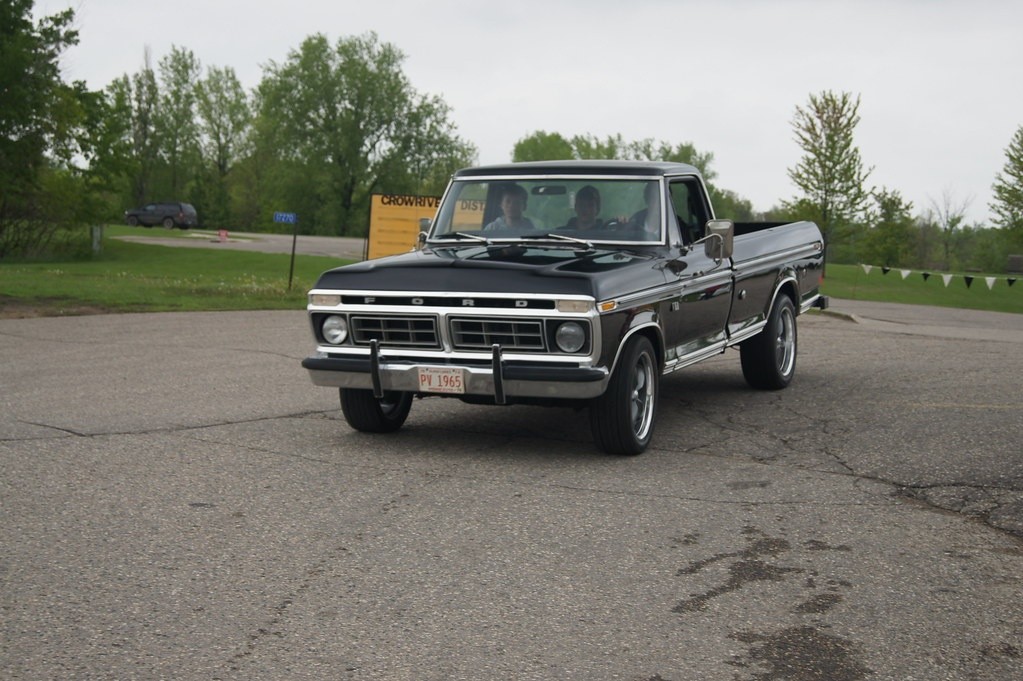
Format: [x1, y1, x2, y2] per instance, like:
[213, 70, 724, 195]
[483, 185, 535, 230]
[615, 182, 689, 247]
[558, 185, 614, 232]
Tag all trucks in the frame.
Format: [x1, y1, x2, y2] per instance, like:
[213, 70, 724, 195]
[301, 159, 829, 456]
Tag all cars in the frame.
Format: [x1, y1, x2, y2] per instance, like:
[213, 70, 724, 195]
[123, 201, 198, 230]
